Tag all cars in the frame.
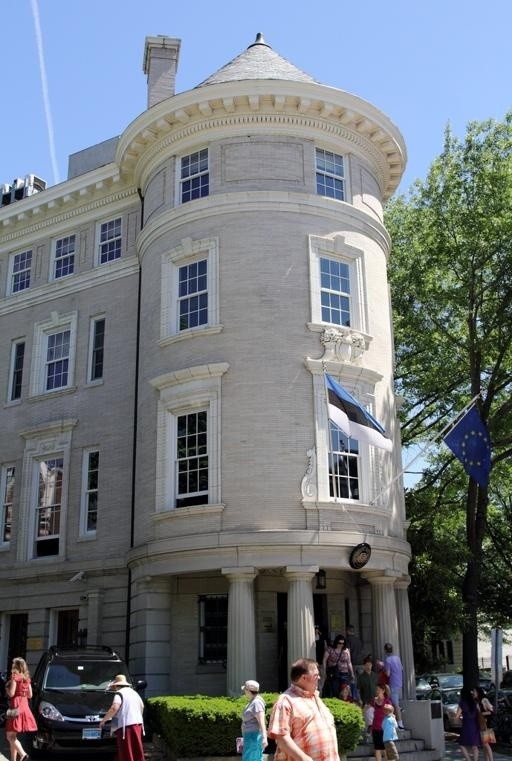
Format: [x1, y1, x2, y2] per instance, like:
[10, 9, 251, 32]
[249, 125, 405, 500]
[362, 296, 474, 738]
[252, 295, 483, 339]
[415, 669, 512, 745]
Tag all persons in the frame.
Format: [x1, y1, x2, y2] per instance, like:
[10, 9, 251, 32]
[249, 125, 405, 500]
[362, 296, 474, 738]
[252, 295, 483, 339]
[241, 679, 269, 761]
[270, 658, 340, 761]
[4, 656, 39, 761]
[100, 675, 146, 761]
[473, 686, 496, 761]
[456, 687, 481, 761]
[314, 624, 405, 761]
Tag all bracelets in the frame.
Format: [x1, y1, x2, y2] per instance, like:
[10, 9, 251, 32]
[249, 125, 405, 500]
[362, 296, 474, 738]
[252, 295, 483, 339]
[4, 685, 9, 688]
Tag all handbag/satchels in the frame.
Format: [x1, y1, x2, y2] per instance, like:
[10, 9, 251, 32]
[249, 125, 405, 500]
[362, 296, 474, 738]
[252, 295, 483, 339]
[326, 664, 340, 679]
[365, 706, 375, 726]
[3, 709, 19, 719]
[480, 729, 496, 744]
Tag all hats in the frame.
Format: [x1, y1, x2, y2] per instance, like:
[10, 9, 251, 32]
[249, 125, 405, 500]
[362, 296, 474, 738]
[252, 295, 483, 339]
[111, 675, 132, 686]
[241, 680, 259, 692]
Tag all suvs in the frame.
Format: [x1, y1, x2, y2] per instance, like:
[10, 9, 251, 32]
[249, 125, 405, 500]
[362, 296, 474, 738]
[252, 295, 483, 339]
[28, 646, 149, 758]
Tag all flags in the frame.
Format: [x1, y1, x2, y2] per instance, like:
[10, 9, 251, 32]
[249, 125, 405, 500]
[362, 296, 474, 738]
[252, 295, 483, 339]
[443, 401, 494, 494]
[326, 373, 393, 451]
